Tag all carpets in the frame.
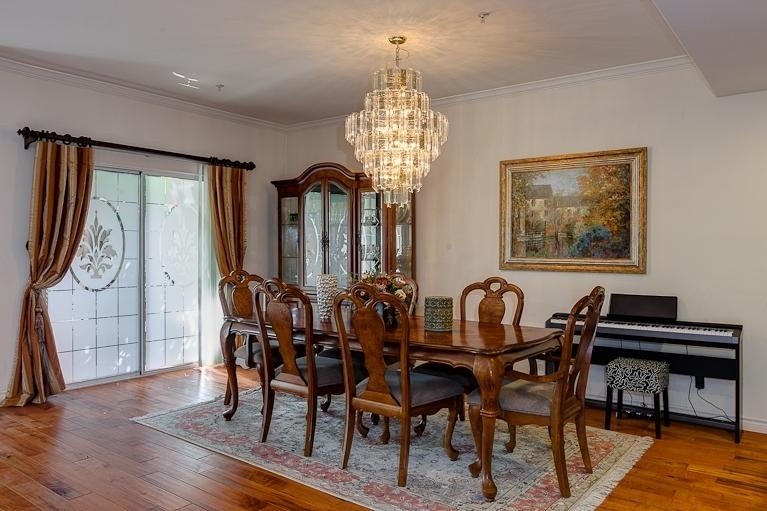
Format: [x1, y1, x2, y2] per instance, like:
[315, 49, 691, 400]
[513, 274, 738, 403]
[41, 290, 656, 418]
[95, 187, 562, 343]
[129, 375, 653, 511]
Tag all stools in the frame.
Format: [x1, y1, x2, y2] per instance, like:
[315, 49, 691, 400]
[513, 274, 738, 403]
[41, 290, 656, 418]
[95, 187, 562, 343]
[602, 357, 671, 442]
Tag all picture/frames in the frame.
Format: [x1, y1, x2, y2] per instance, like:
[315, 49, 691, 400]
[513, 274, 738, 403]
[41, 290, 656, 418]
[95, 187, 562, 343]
[496, 144, 650, 278]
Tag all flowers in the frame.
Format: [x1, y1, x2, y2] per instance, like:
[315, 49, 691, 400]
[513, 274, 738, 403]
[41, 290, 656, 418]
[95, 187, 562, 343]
[373, 271, 410, 308]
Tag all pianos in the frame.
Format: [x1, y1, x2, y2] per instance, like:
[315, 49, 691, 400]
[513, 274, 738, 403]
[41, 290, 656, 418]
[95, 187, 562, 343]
[545, 313, 743, 444]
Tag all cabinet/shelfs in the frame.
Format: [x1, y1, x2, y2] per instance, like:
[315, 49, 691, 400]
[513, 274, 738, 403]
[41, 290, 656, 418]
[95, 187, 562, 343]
[265, 163, 417, 311]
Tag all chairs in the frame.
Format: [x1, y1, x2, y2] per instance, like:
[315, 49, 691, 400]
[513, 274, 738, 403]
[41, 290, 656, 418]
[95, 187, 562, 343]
[413, 277, 524, 452]
[251, 277, 370, 456]
[322, 271, 419, 425]
[468, 286, 605, 498]
[332, 281, 463, 488]
[219, 270, 273, 406]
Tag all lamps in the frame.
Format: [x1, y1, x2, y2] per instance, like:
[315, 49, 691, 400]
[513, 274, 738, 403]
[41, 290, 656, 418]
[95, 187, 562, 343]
[344, 35, 451, 208]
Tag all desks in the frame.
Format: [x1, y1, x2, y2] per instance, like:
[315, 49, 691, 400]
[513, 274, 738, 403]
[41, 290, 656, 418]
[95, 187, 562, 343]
[220, 302, 569, 499]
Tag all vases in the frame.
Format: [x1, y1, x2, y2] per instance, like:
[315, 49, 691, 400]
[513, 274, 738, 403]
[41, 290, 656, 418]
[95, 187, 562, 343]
[383, 308, 398, 332]
